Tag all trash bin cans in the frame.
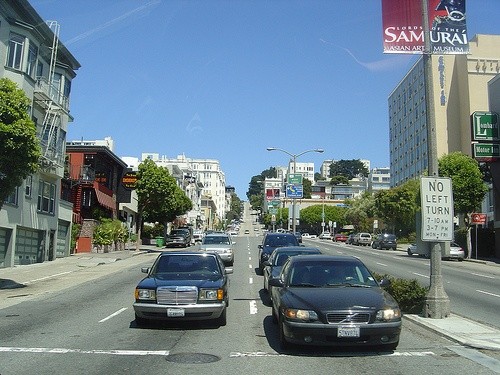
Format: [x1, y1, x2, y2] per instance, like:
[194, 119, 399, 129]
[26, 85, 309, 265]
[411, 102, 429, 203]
[155, 236, 164, 247]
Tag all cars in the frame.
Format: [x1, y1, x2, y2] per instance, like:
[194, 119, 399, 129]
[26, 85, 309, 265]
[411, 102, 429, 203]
[254, 228, 259, 231]
[319, 232, 331, 239]
[245, 230, 249, 234]
[269, 255, 402, 350]
[193, 230, 214, 244]
[408, 238, 464, 261]
[231, 218, 243, 235]
[252, 221, 256, 225]
[133, 250, 233, 326]
[333, 234, 347, 242]
[263, 244, 323, 301]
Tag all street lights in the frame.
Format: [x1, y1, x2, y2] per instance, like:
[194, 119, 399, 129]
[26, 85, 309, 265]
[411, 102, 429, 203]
[257, 181, 274, 232]
[223, 219, 228, 232]
[267, 148, 324, 234]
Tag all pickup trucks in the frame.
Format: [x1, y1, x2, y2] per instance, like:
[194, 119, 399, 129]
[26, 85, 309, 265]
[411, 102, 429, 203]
[302, 233, 317, 238]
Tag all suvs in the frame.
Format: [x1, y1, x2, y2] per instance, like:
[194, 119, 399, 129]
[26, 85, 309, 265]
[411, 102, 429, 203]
[200, 233, 236, 264]
[353, 233, 372, 247]
[166, 228, 192, 248]
[258, 233, 305, 273]
[372, 234, 397, 250]
